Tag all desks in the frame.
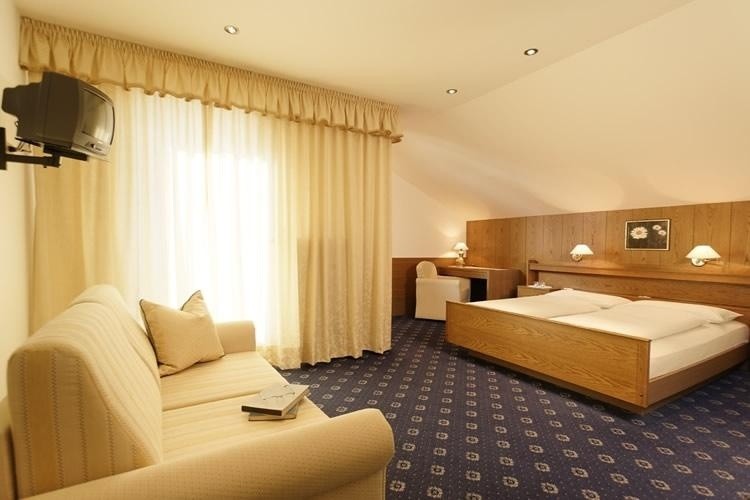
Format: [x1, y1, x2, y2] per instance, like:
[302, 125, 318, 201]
[438, 265, 522, 300]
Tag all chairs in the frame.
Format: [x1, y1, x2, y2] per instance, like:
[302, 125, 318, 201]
[415, 261, 471, 321]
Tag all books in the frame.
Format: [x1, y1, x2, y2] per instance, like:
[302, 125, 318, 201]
[248, 401, 299, 421]
[242, 382, 310, 415]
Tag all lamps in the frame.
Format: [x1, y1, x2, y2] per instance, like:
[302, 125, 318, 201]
[570, 244, 594, 261]
[454, 242, 469, 265]
[685, 245, 721, 266]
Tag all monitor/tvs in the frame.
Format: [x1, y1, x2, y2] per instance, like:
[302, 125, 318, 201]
[1, 72, 115, 162]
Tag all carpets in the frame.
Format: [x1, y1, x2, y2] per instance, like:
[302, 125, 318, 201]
[267, 313, 750, 499]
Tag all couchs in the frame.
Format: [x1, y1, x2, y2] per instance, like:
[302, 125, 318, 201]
[0, 282, 395, 500]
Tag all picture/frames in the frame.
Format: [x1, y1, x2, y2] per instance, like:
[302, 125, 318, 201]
[625, 219, 670, 250]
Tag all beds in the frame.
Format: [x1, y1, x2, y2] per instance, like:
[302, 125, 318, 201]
[446, 260, 750, 416]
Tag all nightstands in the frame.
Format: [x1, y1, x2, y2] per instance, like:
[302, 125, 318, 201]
[517, 285, 552, 296]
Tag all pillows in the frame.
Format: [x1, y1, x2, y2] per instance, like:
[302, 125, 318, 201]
[140, 291, 227, 378]
